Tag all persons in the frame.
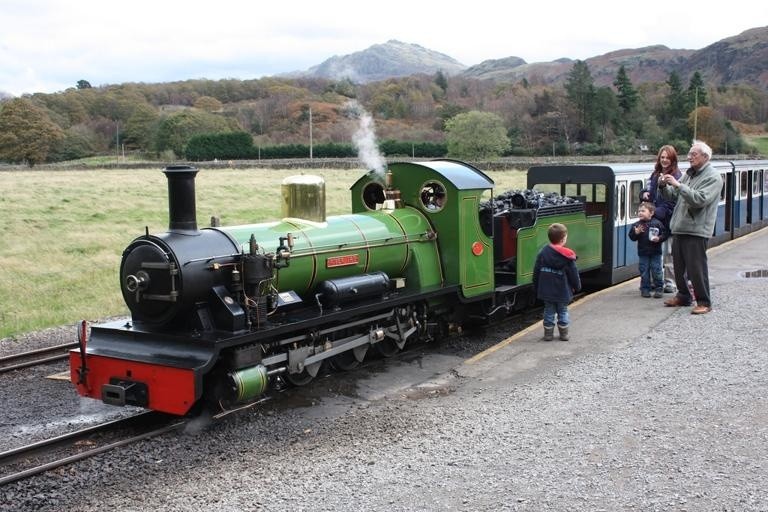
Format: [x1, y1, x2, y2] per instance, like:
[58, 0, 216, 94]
[628, 201, 669, 300]
[642, 144, 686, 292]
[657, 140, 724, 314]
[532, 222, 582, 343]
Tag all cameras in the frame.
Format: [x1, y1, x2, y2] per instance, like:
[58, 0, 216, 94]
[661, 175, 666, 184]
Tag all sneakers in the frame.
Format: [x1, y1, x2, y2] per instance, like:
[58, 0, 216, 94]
[689, 305, 713, 314]
[663, 295, 693, 307]
[641, 289, 663, 298]
[664, 286, 676, 293]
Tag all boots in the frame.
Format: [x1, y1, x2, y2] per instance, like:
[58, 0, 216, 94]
[556, 323, 570, 341]
[543, 326, 555, 341]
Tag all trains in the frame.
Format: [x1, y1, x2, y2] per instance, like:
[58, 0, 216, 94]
[70, 158, 768, 416]
